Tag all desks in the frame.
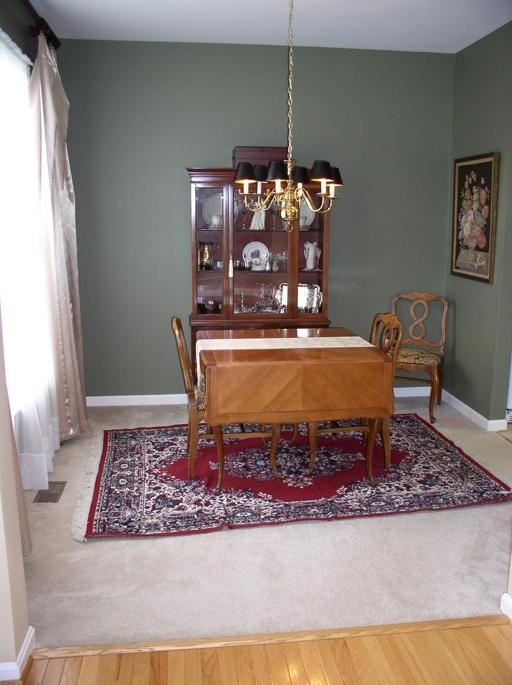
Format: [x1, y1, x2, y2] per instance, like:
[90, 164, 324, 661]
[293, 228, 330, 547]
[192, 324, 394, 495]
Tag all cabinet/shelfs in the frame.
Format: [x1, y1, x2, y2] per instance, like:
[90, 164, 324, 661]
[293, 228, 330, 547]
[186, 164, 333, 389]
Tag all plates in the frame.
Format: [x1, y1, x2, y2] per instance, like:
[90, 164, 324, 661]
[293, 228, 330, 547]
[242, 241, 268, 266]
[282, 200, 316, 225]
[299, 226, 312, 231]
[201, 193, 240, 227]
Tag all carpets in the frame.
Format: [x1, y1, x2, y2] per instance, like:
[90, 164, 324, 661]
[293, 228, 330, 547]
[72, 410, 511, 543]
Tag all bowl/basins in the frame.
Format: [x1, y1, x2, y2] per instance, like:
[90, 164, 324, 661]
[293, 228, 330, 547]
[197, 300, 222, 314]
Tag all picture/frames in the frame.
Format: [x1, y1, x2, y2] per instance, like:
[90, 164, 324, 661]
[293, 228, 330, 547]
[445, 149, 498, 282]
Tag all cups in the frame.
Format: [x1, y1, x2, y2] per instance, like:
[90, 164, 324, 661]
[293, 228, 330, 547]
[207, 215, 223, 230]
[234, 260, 253, 272]
[197, 242, 218, 271]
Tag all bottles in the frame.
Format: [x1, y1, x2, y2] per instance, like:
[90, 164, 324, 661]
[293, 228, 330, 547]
[266, 251, 288, 272]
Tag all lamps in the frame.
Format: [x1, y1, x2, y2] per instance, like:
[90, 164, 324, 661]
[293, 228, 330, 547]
[235, 1, 345, 230]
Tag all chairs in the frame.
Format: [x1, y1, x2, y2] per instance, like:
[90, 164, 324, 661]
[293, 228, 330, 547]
[170, 309, 282, 482]
[307, 312, 405, 473]
[386, 289, 450, 424]
